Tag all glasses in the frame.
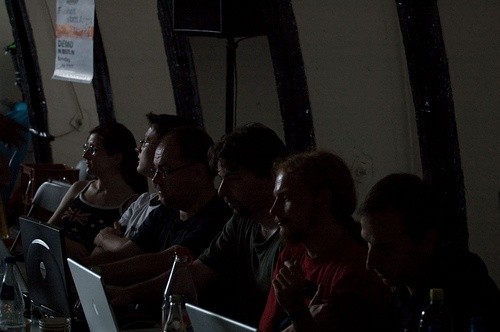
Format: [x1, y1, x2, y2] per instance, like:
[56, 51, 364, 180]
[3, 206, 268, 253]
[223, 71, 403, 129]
[81, 143, 102, 156]
[156, 161, 196, 179]
[140, 138, 157, 147]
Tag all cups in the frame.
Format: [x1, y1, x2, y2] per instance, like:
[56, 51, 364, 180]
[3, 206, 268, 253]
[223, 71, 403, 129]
[39, 317, 72, 332]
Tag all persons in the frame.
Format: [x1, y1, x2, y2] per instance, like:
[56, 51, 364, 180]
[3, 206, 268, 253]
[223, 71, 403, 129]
[94, 124, 233, 280]
[259, 150, 384, 332]
[47, 121, 139, 265]
[271, 173, 500, 332]
[91, 111, 190, 256]
[107, 122, 284, 329]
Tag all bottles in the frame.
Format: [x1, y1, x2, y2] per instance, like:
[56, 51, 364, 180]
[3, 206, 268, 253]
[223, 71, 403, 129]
[164, 294, 193, 332]
[0, 255, 25, 319]
[161, 246, 198, 332]
[418, 289, 454, 332]
[467, 317, 486, 332]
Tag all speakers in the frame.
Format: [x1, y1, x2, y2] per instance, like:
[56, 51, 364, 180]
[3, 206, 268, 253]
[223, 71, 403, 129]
[171, 0, 266, 39]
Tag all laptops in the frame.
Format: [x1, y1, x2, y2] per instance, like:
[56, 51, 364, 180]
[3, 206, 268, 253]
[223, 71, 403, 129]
[66, 258, 163, 332]
[18, 214, 86, 323]
[184, 302, 257, 332]
